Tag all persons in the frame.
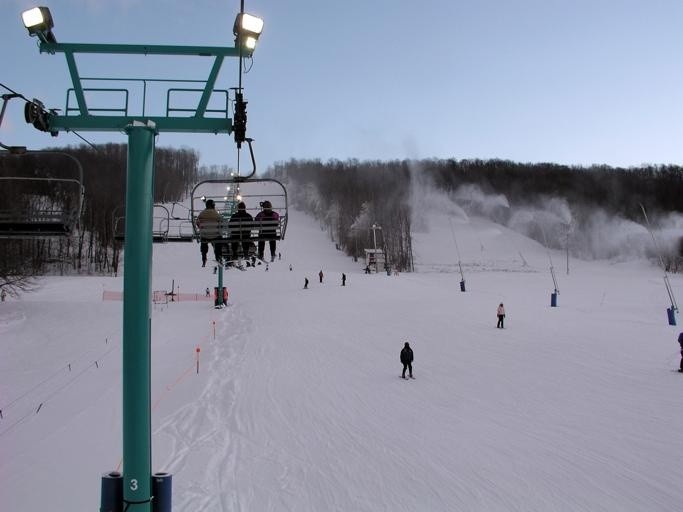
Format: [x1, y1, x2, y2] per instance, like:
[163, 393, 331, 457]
[227, 201, 253, 260]
[400, 342, 413, 379]
[289, 264, 292, 271]
[319, 270, 323, 283]
[254, 200, 280, 262]
[677, 332, 683, 373]
[304, 278, 308, 288]
[341, 273, 346, 286]
[206, 288, 210, 297]
[196, 199, 224, 267]
[496, 302, 505, 328]
[279, 252, 281, 260]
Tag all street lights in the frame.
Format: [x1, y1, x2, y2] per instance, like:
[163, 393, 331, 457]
[368, 224, 381, 273]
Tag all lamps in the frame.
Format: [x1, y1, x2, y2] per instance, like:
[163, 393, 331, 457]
[21, 6, 60, 54]
[232, 10, 264, 52]
